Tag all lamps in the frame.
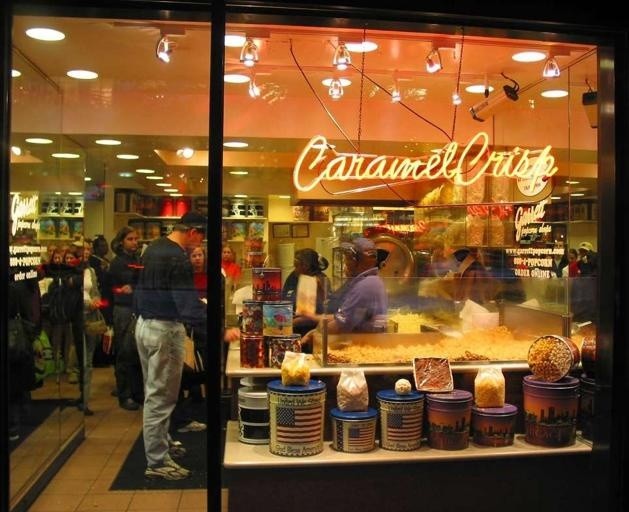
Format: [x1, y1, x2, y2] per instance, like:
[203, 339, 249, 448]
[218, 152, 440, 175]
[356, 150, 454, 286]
[544, 48, 563, 80]
[324, 41, 354, 101]
[154, 25, 179, 65]
[239, 35, 261, 68]
[422, 46, 446, 73]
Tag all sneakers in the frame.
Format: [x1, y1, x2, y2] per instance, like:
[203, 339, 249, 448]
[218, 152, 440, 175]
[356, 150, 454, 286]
[69, 372, 78, 383]
[145, 458, 192, 481]
[167, 440, 186, 457]
[178, 421, 205, 432]
[120, 400, 139, 410]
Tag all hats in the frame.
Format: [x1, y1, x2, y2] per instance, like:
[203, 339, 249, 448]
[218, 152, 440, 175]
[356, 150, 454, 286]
[339, 237, 379, 259]
[578, 242, 593, 253]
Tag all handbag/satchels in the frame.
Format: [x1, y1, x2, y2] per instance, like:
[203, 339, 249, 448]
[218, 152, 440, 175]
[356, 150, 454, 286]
[88, 306, 108, 336]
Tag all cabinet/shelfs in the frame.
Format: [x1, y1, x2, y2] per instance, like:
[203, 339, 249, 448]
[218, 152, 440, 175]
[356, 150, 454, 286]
[220, 340, 597, 511]
[115, 184, 270, 316]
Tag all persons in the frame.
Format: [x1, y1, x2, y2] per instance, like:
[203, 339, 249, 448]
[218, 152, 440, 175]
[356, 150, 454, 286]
[448, 243, 598, 310]
[282, 236, 388, 354]
[2, 207, 240, 430]
[133, 209, 208, 481]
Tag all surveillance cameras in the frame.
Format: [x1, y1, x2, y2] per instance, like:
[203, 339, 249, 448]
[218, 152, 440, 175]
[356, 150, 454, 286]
[582, 91, 597, 128]
[470, 85, 519, 122]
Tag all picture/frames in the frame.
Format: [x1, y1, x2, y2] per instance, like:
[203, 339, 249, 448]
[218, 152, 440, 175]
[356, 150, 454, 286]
[271, 222, 312, 239]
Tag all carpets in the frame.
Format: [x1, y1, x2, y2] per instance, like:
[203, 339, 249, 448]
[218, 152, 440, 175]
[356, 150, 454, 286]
[108, 419, 213, 490]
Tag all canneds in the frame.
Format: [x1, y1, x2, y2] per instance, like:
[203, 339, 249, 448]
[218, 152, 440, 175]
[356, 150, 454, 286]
[262, 299, 294, 338]
[266, 334, 302, 370]
[240, 299, 262, 338]
[238, 336, 266, 369]
[250, 266, 283, 304]
